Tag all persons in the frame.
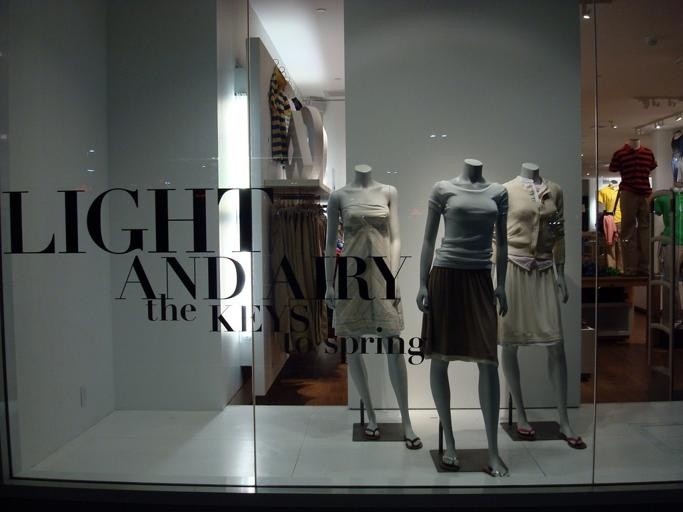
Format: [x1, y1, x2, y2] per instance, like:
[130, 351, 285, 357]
[655, 185, 682, 329]
[490, 164, 587, 450]
[598, 179, 624, 275]
[608, 137, 658, 277]
[415, 157, 509, 478]
[671, 131, 683, 183]
[322, 164, 423, 450]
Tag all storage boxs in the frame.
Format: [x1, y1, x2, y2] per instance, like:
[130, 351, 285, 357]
[581, 326, 597, 367]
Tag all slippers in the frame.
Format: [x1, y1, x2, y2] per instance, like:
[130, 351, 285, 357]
[404, 434, 422, 449]
[364, 427, 380, 440]
[560, 433, 586, 449]
[513, 421, 536, 440]
[440, 456, 459, 471]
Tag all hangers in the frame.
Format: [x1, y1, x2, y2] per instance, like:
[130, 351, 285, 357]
[273, 190, 320, 208]
[272, 58, 293, 95]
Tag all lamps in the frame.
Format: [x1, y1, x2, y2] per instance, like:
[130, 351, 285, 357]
[633, 110, 683, 136]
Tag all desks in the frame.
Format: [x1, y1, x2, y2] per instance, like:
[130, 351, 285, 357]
[581, 272, 662, 348]
